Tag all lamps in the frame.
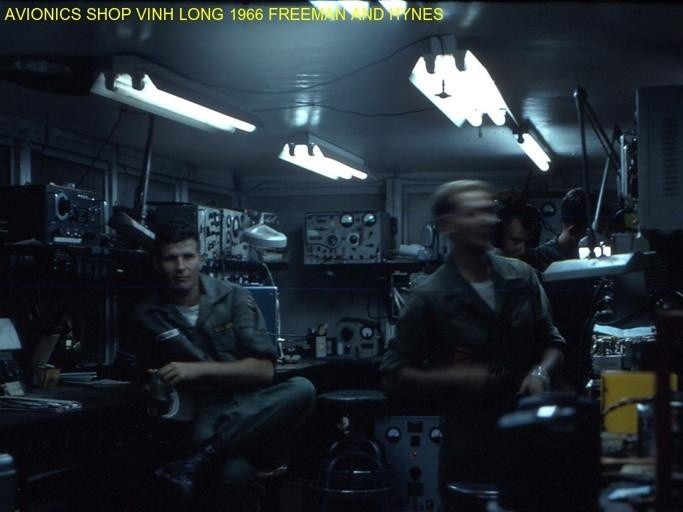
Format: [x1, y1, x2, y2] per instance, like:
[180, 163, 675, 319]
[277, 133, 368, 181]
[408, 48, 552, 171]
[542, 85, 659, 282]
[240, 213, 288, 364]
[89, 56, 259, 134]
[309, 0, 409, 20]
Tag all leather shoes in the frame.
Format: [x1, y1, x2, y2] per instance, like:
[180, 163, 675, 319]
[164, 448, 214, 498]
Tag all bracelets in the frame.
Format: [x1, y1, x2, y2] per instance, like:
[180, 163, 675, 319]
[529, 365, 552, 387]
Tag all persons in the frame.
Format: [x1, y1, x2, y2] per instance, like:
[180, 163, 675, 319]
[126, 216, 321, 508]
[535, 184, 614, 278]
[376, 178, 574, 488]
[484, 187, 543, 261]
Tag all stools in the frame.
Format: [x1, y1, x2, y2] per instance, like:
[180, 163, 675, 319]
[308, 390, 395, 512]
